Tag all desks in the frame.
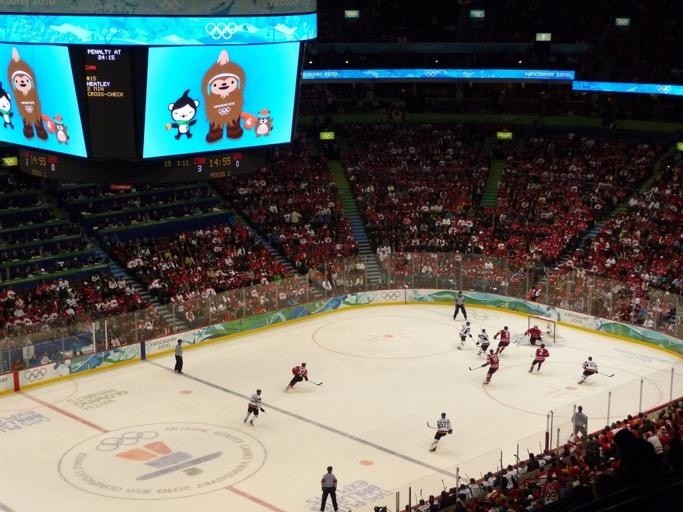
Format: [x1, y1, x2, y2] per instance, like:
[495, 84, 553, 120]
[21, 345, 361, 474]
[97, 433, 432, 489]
[0, 175, 236, 295]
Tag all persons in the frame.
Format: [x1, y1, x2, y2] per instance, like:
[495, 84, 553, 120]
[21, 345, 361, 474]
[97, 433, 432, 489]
[321, 466, 338, 511]
[525, 326, 543, 346]
[529, 344, 549, 372]
[244, 389, 262, 426]
[578, 357, 598, 384]
[453, 291, 467, 320]
[476, 329, 489, 355]
[495, 326, 510, 354]
[406, 399, 682, 511]
[285, 362, 308, 392]
[324, 127, 682, 339]
[484, 350, 499, 384]
[2, 137, 365, 370]
[457, 322, 472, 349]
